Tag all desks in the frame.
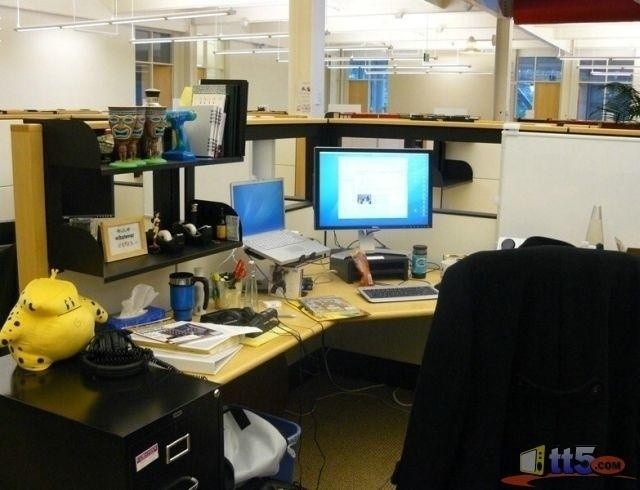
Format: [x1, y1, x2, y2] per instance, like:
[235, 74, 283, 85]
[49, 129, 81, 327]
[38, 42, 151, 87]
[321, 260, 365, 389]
[120, 257, 443, 390]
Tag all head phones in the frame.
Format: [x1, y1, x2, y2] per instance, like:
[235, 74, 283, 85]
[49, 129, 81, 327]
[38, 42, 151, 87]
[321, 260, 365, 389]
[83, 329, 153, 376]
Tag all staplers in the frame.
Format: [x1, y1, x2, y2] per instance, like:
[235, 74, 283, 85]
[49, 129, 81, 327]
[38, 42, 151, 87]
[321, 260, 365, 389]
[246, 308, 281, 338]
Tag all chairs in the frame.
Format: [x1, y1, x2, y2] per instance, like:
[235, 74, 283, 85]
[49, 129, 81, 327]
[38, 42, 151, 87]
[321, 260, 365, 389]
[391, 238, 640, 488]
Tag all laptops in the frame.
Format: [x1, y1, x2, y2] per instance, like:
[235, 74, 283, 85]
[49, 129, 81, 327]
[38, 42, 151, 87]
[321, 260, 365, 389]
[230, 177, 331, 266]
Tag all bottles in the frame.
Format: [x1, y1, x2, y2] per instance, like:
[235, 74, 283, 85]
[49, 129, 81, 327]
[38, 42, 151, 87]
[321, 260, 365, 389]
[193, 267, 206, 316]
[412, 244, 427, 279]
[245, 263, 258, 307]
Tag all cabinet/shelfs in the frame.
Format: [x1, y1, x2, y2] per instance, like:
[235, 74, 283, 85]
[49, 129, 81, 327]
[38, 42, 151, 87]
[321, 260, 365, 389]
[0, 343, 224, 490]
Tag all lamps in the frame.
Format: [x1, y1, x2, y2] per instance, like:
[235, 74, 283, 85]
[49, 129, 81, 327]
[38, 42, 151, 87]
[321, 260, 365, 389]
[13, 2, 640, 76]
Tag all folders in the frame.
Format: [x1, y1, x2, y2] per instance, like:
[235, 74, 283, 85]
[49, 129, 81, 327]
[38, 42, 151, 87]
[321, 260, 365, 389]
[152, 344, 244, 375]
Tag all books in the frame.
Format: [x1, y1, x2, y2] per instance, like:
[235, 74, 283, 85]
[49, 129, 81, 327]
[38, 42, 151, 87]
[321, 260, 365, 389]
[268, 264, 303, 300]
[124, 319, 263, 376]
[170, 79, 248, 159]
[297, 295, 371, 321]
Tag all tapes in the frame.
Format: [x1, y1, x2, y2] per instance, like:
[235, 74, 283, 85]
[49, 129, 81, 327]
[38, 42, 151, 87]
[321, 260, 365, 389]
[182, 223, 196, 235]
[158, 230, 172, 242]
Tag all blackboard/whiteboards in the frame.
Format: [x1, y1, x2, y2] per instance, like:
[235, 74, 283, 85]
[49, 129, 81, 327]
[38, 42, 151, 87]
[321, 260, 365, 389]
[497, 129, 640, 253]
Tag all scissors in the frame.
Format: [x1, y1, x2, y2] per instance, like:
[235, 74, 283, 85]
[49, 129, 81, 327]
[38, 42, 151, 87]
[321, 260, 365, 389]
[230, 259, 247, 288]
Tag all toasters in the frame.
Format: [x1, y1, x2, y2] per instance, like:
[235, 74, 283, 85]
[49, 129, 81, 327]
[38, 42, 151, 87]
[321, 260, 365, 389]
[215, 207, 227, 241]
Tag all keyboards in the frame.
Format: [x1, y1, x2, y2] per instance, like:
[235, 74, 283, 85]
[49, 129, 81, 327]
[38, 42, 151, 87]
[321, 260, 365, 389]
[358, 284, 439, 303]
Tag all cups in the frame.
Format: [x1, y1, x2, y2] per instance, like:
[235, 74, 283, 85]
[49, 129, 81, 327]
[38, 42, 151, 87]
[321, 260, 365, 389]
[169, 272, 209, 322]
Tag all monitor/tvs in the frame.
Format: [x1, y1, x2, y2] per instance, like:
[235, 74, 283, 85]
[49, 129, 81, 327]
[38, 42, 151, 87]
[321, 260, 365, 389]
[313, 146, 433, 259]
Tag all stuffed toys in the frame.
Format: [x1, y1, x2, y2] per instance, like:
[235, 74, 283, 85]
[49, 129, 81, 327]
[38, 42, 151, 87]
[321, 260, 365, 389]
[0, 268, 108, 372]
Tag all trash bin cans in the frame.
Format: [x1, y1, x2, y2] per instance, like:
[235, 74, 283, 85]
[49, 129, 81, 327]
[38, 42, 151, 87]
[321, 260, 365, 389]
[222, 404, 302, 483]
